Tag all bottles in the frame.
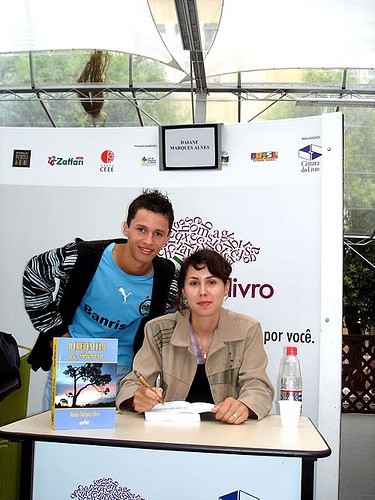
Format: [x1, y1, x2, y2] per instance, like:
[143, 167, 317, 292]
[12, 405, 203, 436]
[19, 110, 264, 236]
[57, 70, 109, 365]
[280, 346, 302, 418]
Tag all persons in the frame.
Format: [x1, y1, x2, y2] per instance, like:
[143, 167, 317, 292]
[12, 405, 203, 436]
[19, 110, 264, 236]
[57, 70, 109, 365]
[20, 187, 181, 412]
[115, 249, 275, 426]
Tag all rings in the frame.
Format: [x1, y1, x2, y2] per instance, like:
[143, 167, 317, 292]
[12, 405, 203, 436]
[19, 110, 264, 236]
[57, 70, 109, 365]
[232, 411, 240, 421]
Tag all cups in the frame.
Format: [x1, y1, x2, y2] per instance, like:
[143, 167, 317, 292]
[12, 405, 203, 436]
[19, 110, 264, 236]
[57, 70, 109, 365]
[278, 400, 302, 427]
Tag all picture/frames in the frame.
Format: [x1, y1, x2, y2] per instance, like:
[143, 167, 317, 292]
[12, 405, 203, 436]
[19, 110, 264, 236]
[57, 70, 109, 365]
[157, 123, 222, 171]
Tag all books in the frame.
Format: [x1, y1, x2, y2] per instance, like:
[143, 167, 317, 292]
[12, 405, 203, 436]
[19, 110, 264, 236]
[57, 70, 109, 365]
[144, 401, 217, 424]
[49, 337, 119, 430]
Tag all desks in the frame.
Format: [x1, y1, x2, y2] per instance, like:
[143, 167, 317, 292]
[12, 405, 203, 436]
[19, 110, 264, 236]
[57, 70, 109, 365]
[0, 409, 333, 500]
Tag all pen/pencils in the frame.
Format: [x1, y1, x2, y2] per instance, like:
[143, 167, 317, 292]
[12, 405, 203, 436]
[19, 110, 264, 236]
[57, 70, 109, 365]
[134, 370, 166, 406]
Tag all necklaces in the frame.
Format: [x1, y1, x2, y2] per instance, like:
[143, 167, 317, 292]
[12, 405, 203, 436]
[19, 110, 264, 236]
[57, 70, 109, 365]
[189, 317, 219, 360]
